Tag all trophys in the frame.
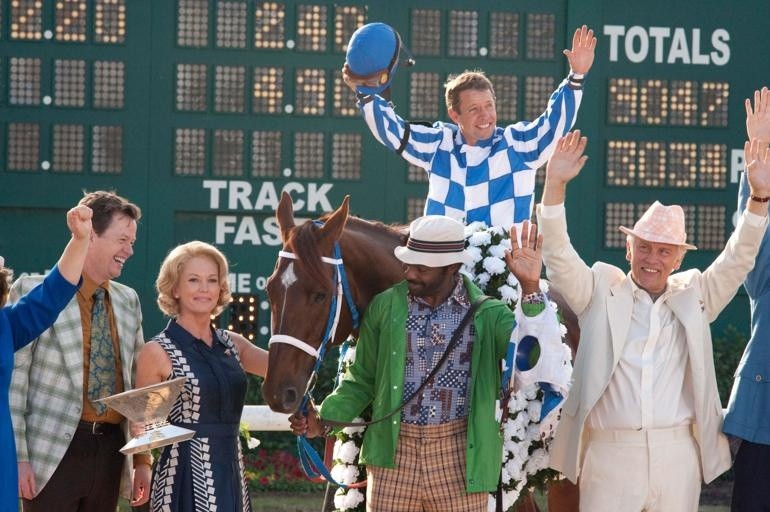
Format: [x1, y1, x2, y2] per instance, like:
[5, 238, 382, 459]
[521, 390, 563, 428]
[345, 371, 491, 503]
[93, 377, 197, 455]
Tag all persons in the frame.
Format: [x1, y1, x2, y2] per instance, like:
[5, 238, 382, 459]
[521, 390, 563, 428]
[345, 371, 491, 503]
[8, 192, 154, 512]
[722, 86, 770, 512]
[130, 241, 269, 512]
[288, 215, 561, 511]
[342, 23, 597, 428]
[0, 205, 94, 512]
[536, 129, 770, 512]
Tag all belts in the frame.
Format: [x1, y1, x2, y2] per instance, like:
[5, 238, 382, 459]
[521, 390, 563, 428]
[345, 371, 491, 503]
[77, 421, 121, 436]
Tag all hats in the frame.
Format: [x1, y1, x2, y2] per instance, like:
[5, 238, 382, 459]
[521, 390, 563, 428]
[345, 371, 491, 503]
[618, 200, 698, 250]
[394, 215, 475, 268]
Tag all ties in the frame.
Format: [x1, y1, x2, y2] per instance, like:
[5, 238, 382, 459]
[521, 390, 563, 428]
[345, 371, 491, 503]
[87, 286, 116, 417]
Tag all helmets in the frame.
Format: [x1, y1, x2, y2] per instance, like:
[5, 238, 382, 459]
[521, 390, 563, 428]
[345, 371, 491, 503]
[339, 21, 405, 95]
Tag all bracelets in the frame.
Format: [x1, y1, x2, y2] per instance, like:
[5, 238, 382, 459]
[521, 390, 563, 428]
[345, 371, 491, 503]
[749, 192, 770, 203]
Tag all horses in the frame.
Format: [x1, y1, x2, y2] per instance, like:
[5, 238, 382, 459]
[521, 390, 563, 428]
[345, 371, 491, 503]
[262, 190, 580, 512]
